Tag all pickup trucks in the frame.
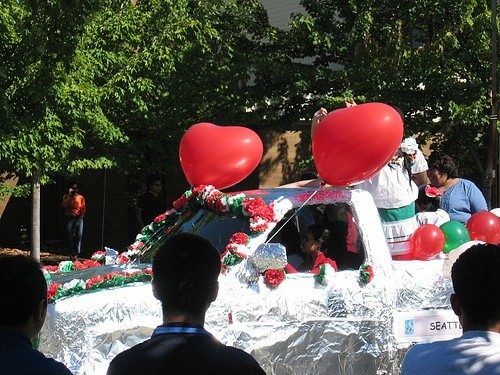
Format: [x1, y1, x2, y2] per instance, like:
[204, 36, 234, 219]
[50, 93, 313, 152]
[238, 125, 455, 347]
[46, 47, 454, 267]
[36, 188, 499, 375]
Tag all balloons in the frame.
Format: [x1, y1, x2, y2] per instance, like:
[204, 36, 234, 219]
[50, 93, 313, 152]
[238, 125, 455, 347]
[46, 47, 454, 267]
[178, 122, 263, 191]
[413, 211, 500, 261]
[312, 101, 404, 188]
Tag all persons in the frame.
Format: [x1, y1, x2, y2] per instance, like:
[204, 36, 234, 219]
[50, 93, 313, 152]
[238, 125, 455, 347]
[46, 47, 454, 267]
[401, 243, 500, 375]
[269, 215, 363, 272]
[107, 231, 266, 374]
[0, 254, 71, 375]
[135, 178, 169, 228]
[62, 180, 87, 263]
[309, 97, 430, 223]
[392, 184, 451, 252]
[427, 151, 489, 224]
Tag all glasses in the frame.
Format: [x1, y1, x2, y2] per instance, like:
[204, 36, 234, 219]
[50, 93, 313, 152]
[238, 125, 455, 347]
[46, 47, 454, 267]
[71, 186, 80, 190]
[302, 235, 316, 243]
